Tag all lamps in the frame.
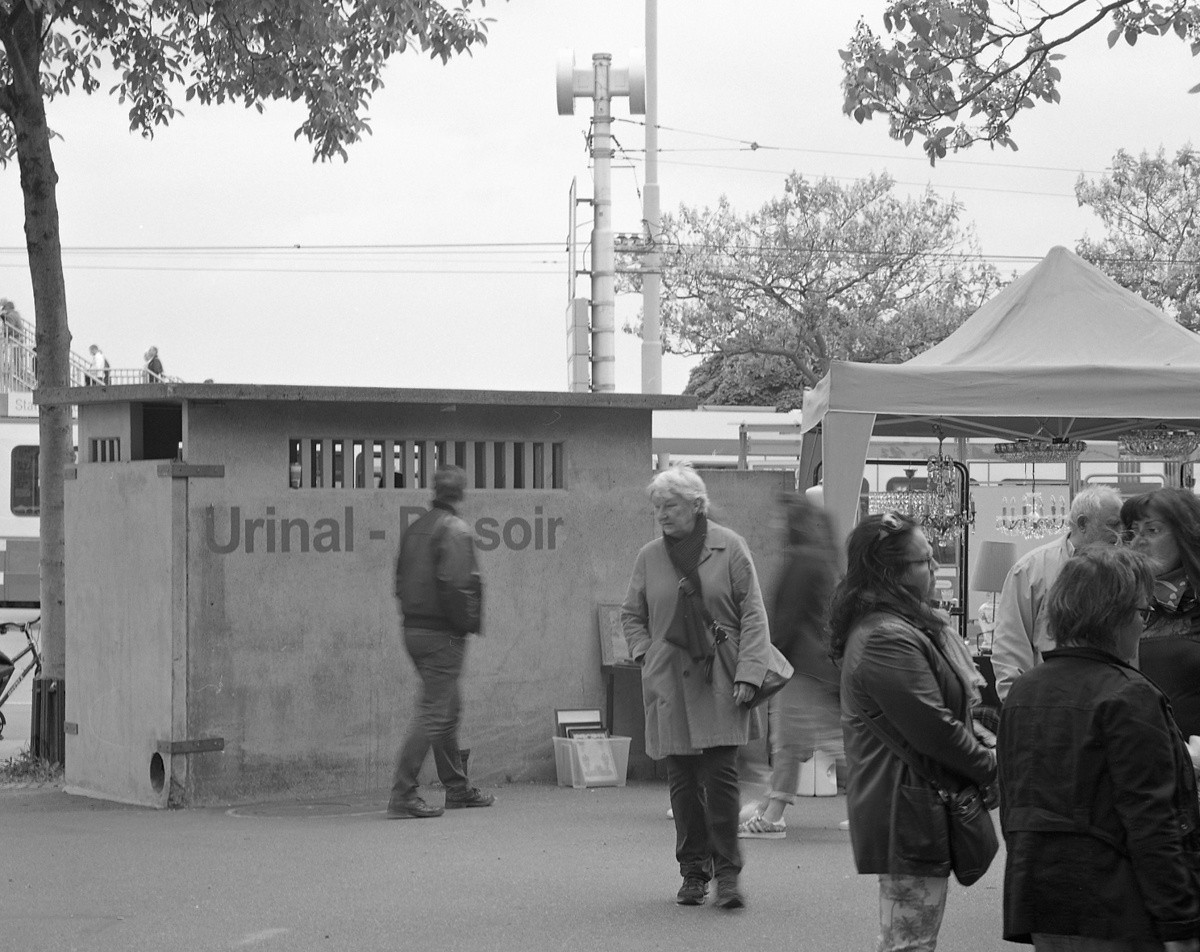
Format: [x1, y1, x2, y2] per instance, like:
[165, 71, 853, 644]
[995, 462, 1070, 540]
[994, 440, 1087, 463]
[970, 542, 1018, 649]
[1117, 424, 1199, 457]
[869, 428, 976, 548]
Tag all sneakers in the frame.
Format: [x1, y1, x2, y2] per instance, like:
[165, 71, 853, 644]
[444, 786, 495, 808]
[676, 877, 711, 906]
[716, 882, 743, 906]
[385, 797, 444, 818]
[738, 812, 786, 839]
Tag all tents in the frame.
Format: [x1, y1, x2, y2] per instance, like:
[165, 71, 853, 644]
[798, 245, 1200, 582]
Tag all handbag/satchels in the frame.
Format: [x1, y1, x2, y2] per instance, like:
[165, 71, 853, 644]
[718, 632, 796, 710]
[948, 784, 999, 887]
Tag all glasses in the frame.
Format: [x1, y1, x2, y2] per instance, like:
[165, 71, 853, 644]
[1134, 604, 1153, 623]
[908, 550, 934, 569]
[870, 512, 904, 553]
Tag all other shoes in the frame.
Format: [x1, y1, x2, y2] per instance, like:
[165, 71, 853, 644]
[840, 820, 851, 831]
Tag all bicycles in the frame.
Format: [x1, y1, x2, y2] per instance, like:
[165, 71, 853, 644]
[0, 615, 41, 735]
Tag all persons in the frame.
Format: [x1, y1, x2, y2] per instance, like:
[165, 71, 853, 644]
[996, 487, 1200, 952]
[384, 465, 494, 817]
[820, 511, 1000, 952]
[621, 457, 771, 909]
[735, 484, 866, 838]
[991, 483, 1123, 702]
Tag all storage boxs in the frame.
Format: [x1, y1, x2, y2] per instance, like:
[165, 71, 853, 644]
[552, 736, 632, 788]
